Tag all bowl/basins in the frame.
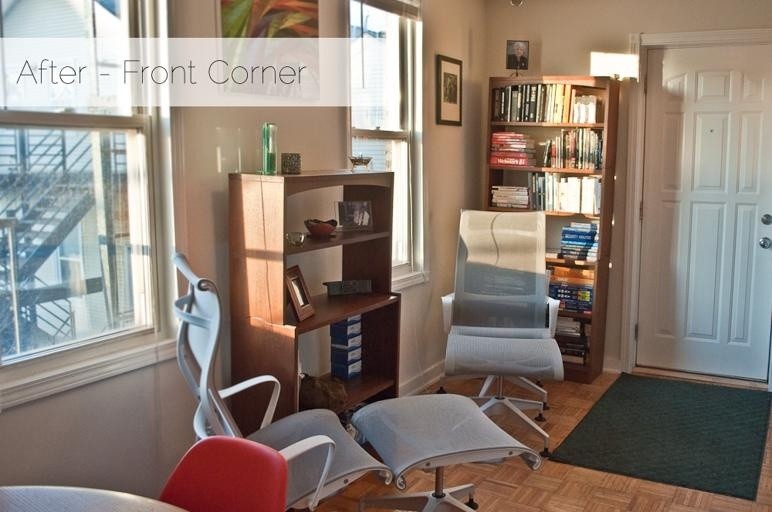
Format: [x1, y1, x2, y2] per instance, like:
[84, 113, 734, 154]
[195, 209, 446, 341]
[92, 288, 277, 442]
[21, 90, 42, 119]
[304, 218, 338, 237]
[285, 232, 308, 247]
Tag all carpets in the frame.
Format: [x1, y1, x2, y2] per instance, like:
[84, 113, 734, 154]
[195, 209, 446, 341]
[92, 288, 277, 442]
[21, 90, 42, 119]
[548, 371, 771, 501]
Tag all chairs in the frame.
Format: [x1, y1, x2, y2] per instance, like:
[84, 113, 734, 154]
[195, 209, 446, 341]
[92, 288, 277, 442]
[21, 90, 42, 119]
[159, 252, 394, 512]
[442, 208, 560, 409]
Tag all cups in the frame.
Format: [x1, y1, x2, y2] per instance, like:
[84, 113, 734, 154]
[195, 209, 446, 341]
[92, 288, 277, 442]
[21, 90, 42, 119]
[281, 152, 301, 175]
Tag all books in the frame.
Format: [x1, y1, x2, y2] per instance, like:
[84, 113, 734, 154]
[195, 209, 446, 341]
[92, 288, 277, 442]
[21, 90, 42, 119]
[546, 221, 599, 364]
[490, 83, 602, 215]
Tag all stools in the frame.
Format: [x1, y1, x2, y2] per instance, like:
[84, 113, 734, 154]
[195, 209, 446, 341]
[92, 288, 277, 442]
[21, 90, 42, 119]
[350, 393, 541, 512]
[445, 333, 564, 457]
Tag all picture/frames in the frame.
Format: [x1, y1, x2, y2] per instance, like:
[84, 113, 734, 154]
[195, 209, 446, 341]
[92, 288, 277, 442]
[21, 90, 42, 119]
[333, 200, 374, 232]
[435, 53, 463, 127]
[505, 40, 529, 70]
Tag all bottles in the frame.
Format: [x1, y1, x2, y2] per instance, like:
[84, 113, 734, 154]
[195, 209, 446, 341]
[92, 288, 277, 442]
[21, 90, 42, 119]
[262, 120, 277, 176]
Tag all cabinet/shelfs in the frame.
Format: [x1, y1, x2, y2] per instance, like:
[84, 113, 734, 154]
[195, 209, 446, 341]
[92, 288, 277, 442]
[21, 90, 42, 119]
[227, 171, 402, 448]
[483, 75, 620, 385]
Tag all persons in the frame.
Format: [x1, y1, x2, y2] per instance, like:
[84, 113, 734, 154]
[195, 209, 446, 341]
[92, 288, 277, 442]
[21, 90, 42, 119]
[507, 42, 528, 69]
[354, 205, 369, 226]
[446, 75, 457, 104]
